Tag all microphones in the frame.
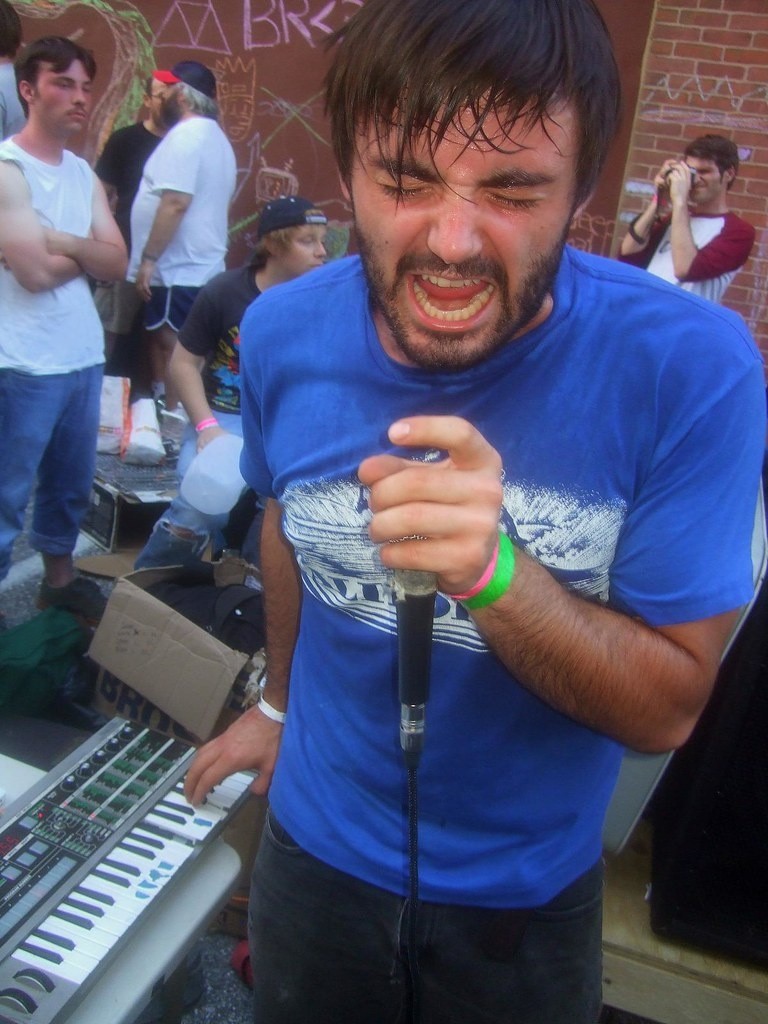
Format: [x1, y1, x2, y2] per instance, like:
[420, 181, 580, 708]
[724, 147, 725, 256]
[388, 535, 439, 771]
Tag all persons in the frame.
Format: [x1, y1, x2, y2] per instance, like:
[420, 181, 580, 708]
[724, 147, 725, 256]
[183, 0, 768, 1024]
[0, 0, 326, 623]
[618, 134, 756, 304]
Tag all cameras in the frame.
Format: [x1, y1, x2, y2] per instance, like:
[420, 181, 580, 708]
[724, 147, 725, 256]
[666, 166, 697, 188]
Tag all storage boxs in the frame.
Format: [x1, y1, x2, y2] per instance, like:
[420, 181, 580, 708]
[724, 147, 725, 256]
[83, 476, 178, 554]
[70, 545, 267, 748]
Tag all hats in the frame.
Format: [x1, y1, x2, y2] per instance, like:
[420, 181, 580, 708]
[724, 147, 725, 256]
[258, 197, 328, 240]
[152, 61, 216, 99]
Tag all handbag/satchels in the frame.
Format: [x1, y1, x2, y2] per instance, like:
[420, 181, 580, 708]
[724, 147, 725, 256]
[121, 399, 167, 466]
[96, 375, 131, 454]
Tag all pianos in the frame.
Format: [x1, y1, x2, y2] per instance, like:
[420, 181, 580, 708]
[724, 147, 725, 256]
[0, 646, 271, 1024]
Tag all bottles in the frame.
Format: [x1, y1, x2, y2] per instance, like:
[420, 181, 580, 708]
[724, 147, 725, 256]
[181, 434, 247, 514]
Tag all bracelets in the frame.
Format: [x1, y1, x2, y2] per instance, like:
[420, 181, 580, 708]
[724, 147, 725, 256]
[195, 418, 219, 433]
[450, 530, 515, 611]
[142, 252, 158, 261]
[258, 696, 286, 724]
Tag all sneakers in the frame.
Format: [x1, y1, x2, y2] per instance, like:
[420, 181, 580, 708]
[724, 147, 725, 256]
[36, 577, 109, 628]
[162, 436, 180, 461]
[150, 390, 167, 422]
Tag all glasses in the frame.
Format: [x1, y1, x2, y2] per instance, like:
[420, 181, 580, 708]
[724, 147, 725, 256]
[151, 92, 165, 101]
